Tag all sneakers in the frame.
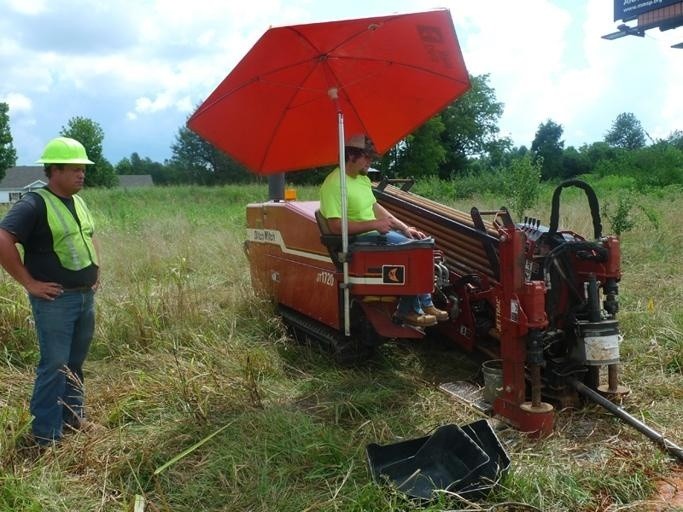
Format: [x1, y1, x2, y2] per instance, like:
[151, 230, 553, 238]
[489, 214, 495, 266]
[79, 419, 106, 434]
[423, 304, 449, 322]
[391, 308, 439, 328]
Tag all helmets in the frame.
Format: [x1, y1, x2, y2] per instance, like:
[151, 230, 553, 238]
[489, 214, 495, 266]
[32, 134, 95, 171]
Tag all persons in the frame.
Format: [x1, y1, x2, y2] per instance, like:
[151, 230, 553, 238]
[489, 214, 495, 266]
[316, 132, 450, 327]
[0, 137, 109, 446]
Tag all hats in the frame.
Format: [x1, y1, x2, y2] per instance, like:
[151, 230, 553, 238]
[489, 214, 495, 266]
[344, 134, 381, 157]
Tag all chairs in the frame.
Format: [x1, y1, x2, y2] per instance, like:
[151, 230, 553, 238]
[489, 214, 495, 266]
[315, 209, 388, 271]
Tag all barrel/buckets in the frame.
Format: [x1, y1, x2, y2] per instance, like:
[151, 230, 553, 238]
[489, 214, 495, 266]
[482, 359, 504, 404]
[577, 318, 619, 368]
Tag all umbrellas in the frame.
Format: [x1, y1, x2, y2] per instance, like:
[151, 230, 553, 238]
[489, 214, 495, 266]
[184, 7, 472, 337]
